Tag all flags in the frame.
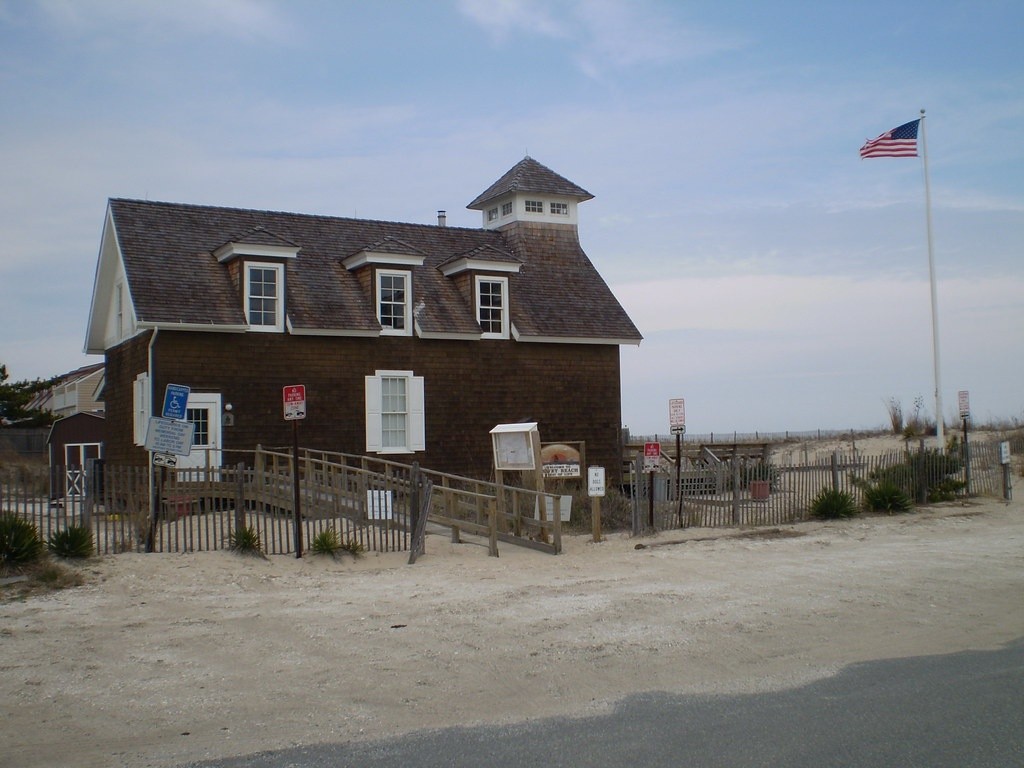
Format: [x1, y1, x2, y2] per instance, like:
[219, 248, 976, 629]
[860, 114, 925, 159]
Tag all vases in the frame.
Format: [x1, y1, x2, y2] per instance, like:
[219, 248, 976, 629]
[751, 481, 770, 499]
